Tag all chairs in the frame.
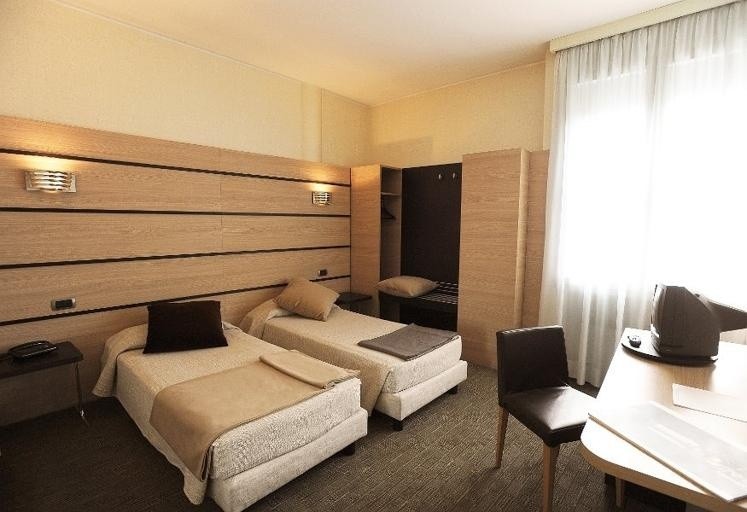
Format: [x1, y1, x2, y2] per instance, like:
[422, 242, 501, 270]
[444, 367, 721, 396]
[491, 323, 627, 512]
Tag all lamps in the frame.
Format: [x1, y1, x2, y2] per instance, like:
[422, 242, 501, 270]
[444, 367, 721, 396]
[311, 190, 333, 208]
[22, 169, 77, 194]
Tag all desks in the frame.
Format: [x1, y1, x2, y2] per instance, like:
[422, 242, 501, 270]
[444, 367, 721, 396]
[0, 340, 91, 430]
[376, 283, 458, 324]
[574, 325, 746, 511]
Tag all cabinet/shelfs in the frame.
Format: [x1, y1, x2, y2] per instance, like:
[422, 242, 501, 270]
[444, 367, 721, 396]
[347, 162, 405, 323]
[454, 147, 532, 373]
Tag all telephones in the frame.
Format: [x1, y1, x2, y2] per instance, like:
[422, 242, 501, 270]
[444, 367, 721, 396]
[8, 340, 57, 360]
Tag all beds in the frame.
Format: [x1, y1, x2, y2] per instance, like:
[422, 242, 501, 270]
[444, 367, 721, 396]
[101, 317, 370, 511]
[240, 295, 469, 431]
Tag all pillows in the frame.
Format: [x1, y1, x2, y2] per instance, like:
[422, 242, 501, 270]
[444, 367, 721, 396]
[373, 273, 441, 300]
[141, 297, 230, 355]
[272, 273, 341, 322]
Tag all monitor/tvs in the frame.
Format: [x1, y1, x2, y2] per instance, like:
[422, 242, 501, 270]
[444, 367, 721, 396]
[652, 282, 747, 358]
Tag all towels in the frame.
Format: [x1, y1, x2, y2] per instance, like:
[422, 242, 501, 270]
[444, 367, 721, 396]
[355, 320, 461, 364]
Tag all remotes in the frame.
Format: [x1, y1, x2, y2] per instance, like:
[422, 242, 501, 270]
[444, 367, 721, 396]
[628, 334, 642, 346]
